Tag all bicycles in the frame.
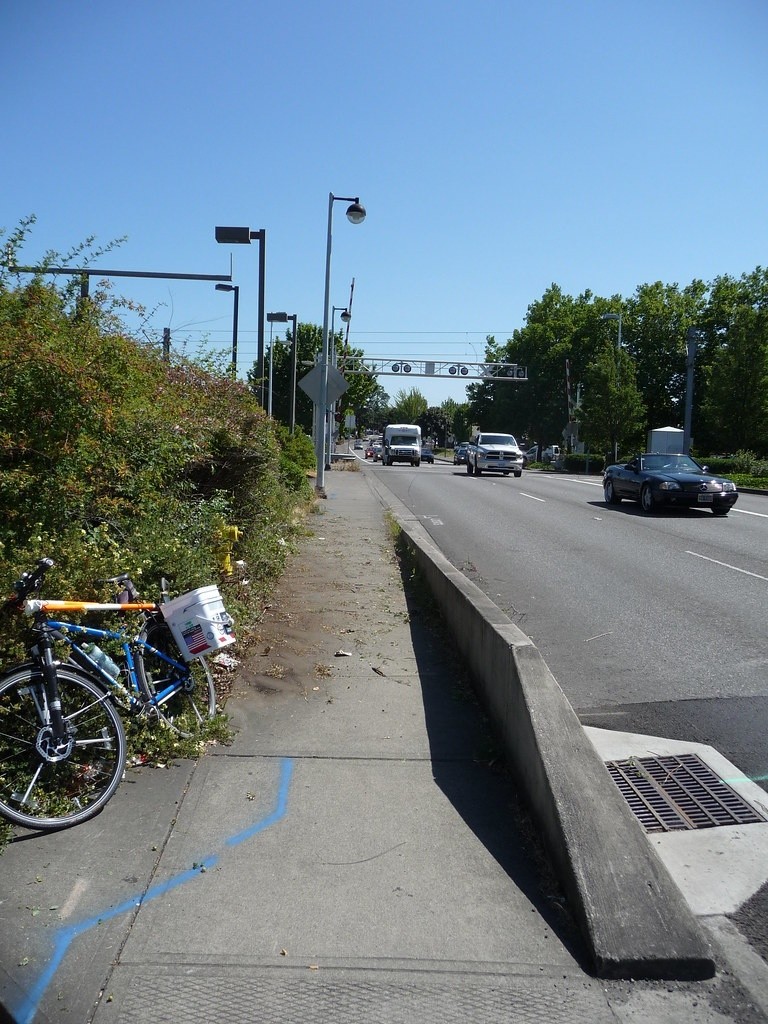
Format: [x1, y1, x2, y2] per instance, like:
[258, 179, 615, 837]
[0, 557, 217, 832]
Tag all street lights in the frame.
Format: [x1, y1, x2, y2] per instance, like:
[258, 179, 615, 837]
[312, 190, 368, 502]
[215, 224, 265, 407]
[266, 312, 298, 437]
[600, 312, 624, 464]
[215, 282, 239, 381]
[324, 304, 353, 471]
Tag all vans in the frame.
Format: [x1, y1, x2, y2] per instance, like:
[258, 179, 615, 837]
[523, 445, 560, 462]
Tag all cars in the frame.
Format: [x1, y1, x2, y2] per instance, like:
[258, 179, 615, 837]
[421, 449, 435, 464]
[454, 448, 467, 465]
[354, 432, 383, 462]
[602, 452, 739, 516]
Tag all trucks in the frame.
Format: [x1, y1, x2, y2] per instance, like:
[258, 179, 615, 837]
[381, 424, 422, 467]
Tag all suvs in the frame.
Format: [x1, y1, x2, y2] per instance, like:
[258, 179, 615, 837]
[467, 432, 524, 477]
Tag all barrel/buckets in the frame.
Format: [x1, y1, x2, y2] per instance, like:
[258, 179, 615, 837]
[157, 585, 237, 663]
